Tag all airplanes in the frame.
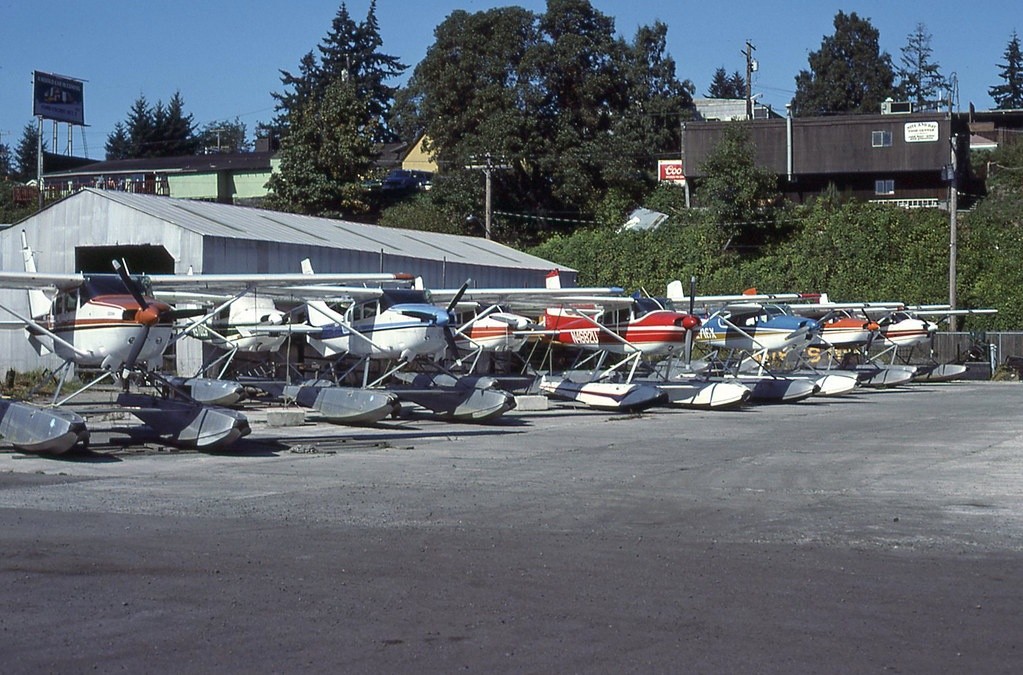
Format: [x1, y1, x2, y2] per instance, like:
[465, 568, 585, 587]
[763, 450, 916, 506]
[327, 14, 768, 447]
[0, 228, 1000, 462]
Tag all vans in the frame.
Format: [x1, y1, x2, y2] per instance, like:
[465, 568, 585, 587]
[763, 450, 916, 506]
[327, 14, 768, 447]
[382, 168, 435, 199]
